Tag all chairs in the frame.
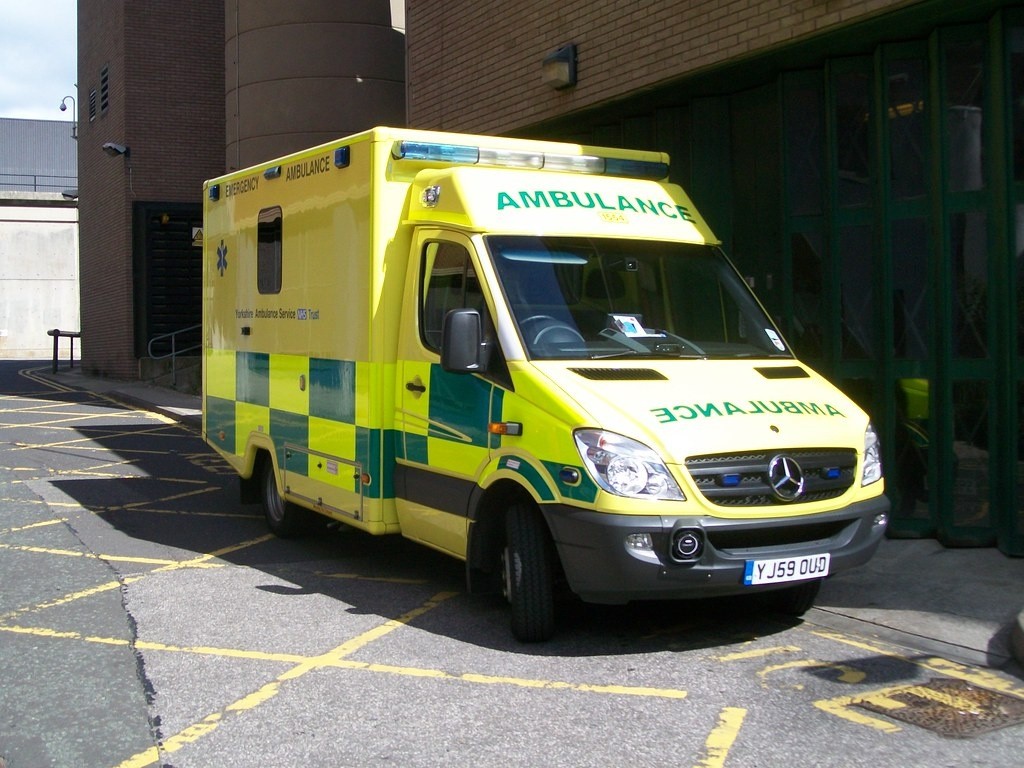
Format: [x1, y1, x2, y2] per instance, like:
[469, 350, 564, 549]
[586, 267, 626, 339]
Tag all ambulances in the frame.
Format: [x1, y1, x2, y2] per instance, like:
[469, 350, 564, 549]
[200, 128, 890, 644]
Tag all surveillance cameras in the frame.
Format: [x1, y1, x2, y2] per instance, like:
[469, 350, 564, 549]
[60, 103, 66, 111]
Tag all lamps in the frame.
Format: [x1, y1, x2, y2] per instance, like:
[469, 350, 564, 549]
[61, 191, 79, 201]
[103, 142, 130, 157]
[61, 96, 80, 139]
[540, 44, 576, 91]
